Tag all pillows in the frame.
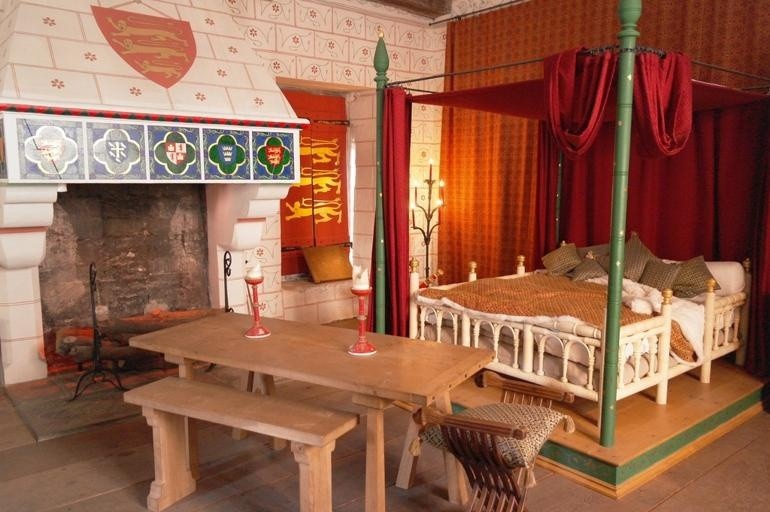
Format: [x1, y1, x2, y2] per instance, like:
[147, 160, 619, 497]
[410, 402, 575, 487]
[542, 231, 722, 299]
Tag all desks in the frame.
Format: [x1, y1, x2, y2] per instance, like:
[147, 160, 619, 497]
[128, 310, 496, 506]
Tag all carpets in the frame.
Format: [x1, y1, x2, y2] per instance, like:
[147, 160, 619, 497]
[4, 358, 236, 444]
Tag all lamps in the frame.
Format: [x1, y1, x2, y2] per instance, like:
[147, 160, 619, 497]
[411, 156, 448, 287]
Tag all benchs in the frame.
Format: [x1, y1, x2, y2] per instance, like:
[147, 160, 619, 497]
[124, 375, 360, 512]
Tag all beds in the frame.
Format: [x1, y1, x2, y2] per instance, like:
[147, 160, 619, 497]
[409, 240, 752, 428]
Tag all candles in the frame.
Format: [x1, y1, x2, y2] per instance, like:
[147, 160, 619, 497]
[245, 261, 264, 279]
[352, 264, 370, 291]
